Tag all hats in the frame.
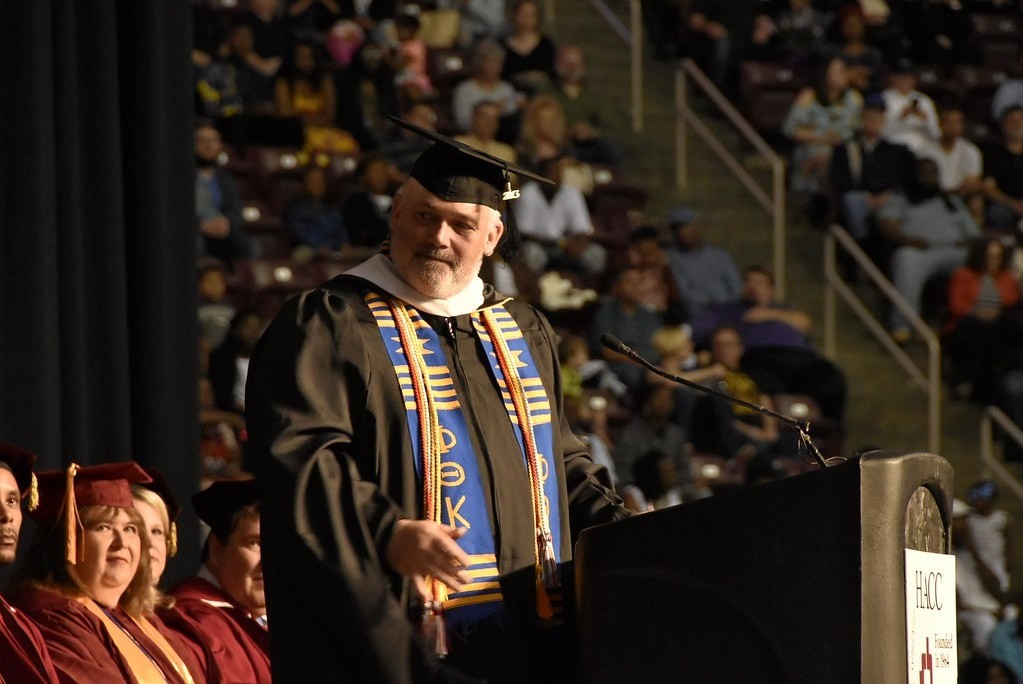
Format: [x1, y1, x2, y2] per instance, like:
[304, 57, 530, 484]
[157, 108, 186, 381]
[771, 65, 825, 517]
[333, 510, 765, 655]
[29, 461, 153, 565]
[386, 113, 557, 211]
[0, 442, 39, 513]
[193, 476, 261, 528]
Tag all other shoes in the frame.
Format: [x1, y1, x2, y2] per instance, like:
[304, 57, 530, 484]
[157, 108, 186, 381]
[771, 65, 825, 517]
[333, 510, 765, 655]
[891, 327, 914, 345]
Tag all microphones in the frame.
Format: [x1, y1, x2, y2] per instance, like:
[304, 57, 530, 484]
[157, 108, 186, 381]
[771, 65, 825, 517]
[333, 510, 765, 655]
[599, 334, 831, 470]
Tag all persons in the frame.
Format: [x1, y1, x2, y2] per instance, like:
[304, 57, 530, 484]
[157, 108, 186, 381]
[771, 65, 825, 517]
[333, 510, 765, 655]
[245, 117, 646, 684]
[0, 0, 846, 684]
[951, 481, 1023, 684]
[616, 0, 1023, 461]
